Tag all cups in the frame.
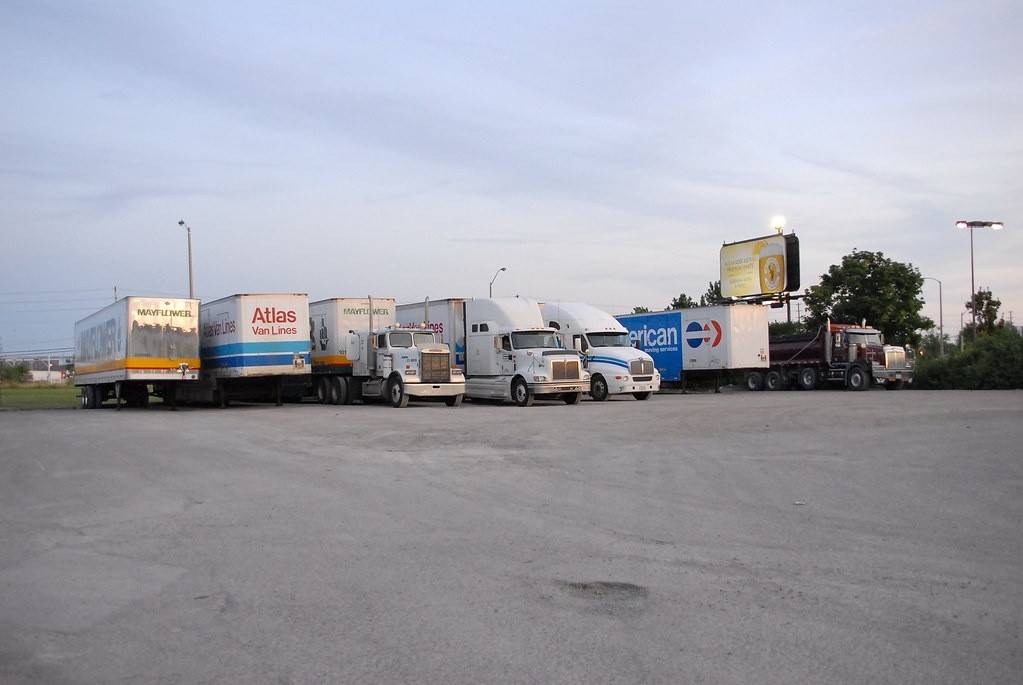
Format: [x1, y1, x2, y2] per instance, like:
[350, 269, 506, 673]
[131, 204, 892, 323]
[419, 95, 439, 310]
[759, 245, 784, 293]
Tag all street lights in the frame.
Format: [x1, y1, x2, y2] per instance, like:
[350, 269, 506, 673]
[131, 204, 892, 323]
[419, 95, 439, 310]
[489, 268, 505, 297]
[921, 277, 944, 357]
[957, 221, 1004, 341]
[178, 219, 193, 299]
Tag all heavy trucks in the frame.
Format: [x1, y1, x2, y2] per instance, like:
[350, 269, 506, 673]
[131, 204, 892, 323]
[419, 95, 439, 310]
[309, 295, 467, 408]
[738, 317, 916, 392]
[537, 299, 661, 402]
[396, 296, 590, 408]
